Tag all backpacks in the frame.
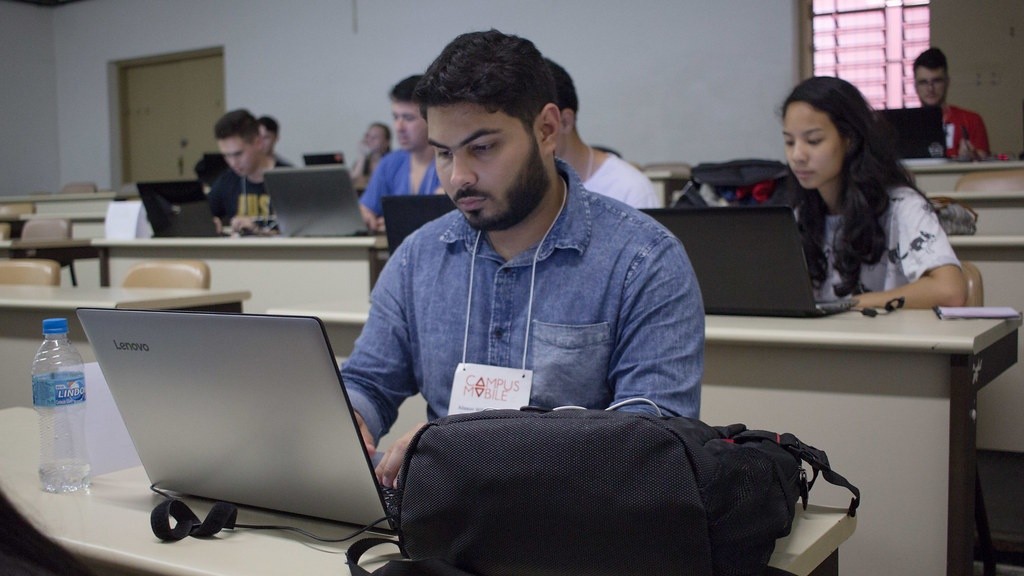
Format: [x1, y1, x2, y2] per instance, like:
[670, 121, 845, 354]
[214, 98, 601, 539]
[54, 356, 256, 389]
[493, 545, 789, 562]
[345, 405, 860, 576]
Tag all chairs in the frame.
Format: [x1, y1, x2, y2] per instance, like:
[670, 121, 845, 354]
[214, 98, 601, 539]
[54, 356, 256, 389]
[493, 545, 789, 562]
[958, 263, 997, 576]
[0, 203, 37, 216]
[0, 258, 61, 288]
[60, 183, 97, 193]
[121, 258, 211, 290]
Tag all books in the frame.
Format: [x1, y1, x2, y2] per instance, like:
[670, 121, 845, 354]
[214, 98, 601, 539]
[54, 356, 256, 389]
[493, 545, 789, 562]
[940, 307, 1020, 318]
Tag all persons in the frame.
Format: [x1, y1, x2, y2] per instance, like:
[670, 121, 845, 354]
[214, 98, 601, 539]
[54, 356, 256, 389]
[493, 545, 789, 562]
[758, 75, 969, 309]
[359, 75, 447, 235]
[543, 57, 662, 209]
[351, 121, 392, 181]
[206, 108, 296, 237]
[338, 26, 704, 489]
[913, 46, 991, 161]
[256, 115, 280, 156]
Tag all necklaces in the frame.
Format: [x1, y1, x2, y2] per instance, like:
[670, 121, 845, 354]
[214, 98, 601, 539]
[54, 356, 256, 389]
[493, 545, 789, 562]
[585, 143, 594, 182]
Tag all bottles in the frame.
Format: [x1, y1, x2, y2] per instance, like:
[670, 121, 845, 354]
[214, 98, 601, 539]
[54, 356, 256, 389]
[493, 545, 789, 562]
[31, 317, 92, 492]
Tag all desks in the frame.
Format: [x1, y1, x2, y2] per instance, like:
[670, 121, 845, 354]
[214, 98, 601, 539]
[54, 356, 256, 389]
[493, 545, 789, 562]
[0, 159, 1024, 576]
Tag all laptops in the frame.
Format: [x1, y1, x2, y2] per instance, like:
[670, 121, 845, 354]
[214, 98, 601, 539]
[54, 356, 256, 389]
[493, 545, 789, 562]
[878, 106, 948, 160]
[640, 204, 860, 319]
[262, 152, 459, 255]
[136, 180, 226, 237]
[76, 306, 402, 541]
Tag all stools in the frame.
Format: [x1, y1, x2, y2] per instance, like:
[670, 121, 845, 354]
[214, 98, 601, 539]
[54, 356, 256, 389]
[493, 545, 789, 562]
[22, 218, 78, 287]
